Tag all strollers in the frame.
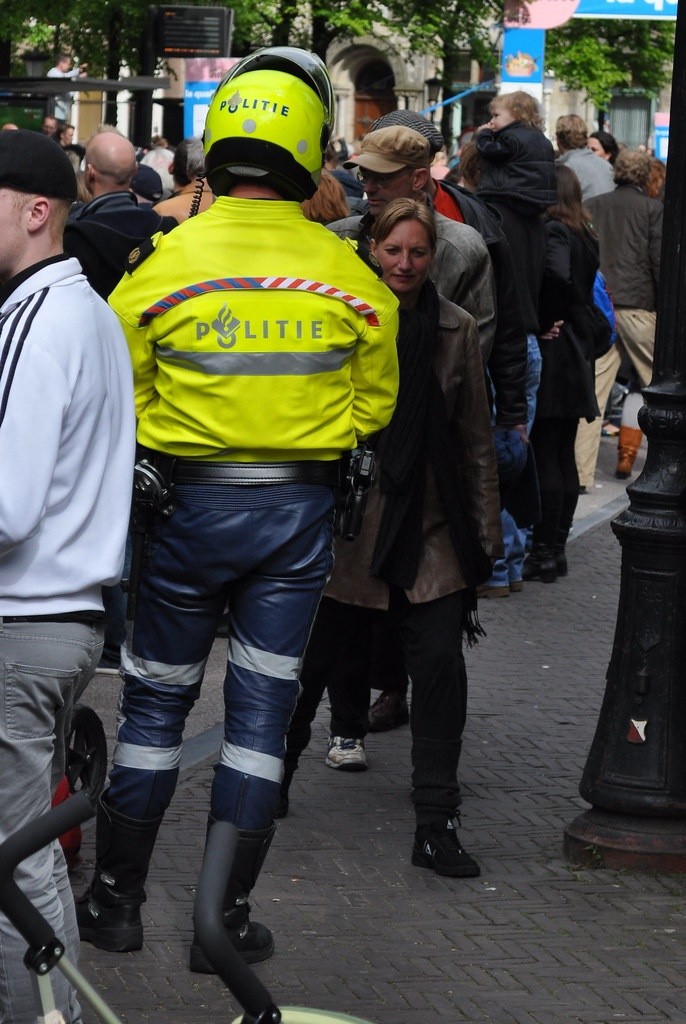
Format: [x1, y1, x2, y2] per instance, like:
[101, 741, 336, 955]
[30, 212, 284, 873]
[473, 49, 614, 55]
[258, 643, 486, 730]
[0, 790, 374, 1024]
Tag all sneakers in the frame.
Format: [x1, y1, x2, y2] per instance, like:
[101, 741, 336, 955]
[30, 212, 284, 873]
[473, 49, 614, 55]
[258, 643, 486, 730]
[412, 809, 480, 877]
[368, 690, 408, 726]
[326, 736, 368, 769]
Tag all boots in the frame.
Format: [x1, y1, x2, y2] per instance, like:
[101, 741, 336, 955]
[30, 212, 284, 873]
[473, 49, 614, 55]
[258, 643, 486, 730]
[189, 814, 274, 974]
[555, 529, 568, 576]
[616, 425, 643, 477]
[74, 788, 164, 952]
[521, 541, 556, 583]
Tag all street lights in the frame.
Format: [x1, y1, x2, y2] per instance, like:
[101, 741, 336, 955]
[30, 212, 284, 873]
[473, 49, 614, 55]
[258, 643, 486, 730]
[425, 78, 442, 124]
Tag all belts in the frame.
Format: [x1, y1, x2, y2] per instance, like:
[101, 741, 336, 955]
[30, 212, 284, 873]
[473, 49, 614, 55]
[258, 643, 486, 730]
[156, 459, 339, 485]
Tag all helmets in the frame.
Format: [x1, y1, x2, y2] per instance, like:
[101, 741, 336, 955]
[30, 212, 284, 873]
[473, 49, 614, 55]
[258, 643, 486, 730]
[205, 56, 329, 199]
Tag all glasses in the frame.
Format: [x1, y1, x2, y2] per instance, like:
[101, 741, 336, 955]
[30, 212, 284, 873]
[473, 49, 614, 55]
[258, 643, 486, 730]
[356, 171, 414, 187]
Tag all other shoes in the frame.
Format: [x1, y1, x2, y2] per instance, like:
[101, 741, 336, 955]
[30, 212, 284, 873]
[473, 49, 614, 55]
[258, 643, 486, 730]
[602, 423, 619, 435]
[94, 658, 120, 674]
[476, 584, 510, 598]
[509, 580, 523, 591]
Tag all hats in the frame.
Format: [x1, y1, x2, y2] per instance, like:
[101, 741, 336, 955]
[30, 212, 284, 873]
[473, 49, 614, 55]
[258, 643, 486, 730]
[0, 129, 78, 201]
[343, 124, 431, 174]
[130, 164, 163, 202]
[370, 110, 444, 153]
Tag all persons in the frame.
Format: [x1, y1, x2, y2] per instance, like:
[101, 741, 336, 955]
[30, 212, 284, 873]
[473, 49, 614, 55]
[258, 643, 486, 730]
[1, 127, 134, 1024]
[323, 200, 500, 879]
[1, 48, 669, 1024]
[103, 48, 398, 974]
